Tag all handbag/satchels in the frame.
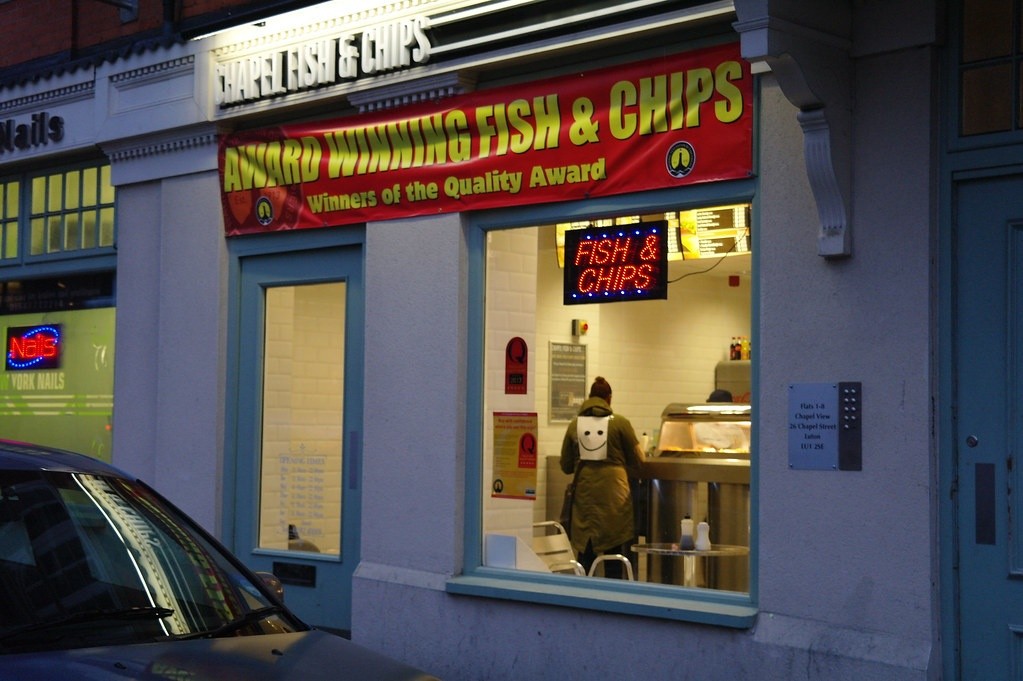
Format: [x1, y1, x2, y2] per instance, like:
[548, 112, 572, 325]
[557, 484, 574, 541]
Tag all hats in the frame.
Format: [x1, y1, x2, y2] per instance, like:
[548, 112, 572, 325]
[707, 390, 731, 403]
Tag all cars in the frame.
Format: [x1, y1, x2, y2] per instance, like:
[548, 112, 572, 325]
[0, 440, 440, 681]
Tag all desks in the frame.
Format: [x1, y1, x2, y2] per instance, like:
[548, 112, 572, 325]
[631, 543, 749, 589]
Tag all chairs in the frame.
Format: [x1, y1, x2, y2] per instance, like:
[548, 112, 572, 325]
[532, 521, 634, 581]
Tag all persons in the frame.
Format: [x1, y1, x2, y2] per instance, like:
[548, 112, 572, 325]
[706, 389, 732, 402]
[560, 376, 645, 579]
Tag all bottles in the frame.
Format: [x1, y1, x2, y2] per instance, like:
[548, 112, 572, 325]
[695, 522, 711, 551]
[730, 337, 735, 360]
[741, 335, 750, 360]
[680, 512, 695, 550]
[736, 336, 741, 359]
[641, 432, 649, 451]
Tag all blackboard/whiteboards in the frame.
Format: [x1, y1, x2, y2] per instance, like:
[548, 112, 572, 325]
[549, 341, 586, 424]
